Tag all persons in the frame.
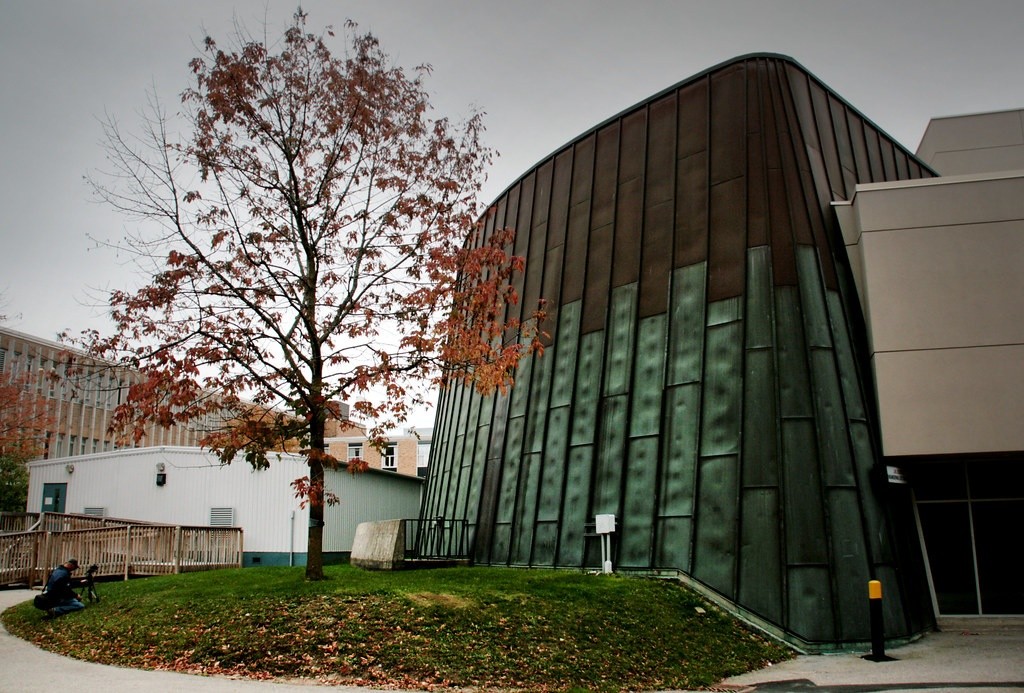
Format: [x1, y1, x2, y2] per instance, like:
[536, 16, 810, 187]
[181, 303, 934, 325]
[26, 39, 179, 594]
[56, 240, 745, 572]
[45, 559, 84, 615]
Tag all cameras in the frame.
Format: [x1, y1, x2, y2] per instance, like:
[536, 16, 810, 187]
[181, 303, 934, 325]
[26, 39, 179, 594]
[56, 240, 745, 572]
[85, 564, 99, 575]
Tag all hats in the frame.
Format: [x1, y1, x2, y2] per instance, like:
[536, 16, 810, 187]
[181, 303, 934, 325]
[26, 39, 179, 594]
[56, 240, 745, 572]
[69, 558, 80, 570]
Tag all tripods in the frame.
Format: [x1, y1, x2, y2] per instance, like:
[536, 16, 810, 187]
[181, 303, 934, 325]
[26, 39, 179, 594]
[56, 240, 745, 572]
[80, 573, 100, 605]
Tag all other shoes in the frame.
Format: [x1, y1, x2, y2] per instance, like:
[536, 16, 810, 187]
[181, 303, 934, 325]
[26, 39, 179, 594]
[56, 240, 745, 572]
[47, 608, 56, 621]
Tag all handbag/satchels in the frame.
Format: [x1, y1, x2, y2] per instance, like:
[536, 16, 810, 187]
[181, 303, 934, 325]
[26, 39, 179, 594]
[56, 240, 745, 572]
[35, 593, 53, 609]
